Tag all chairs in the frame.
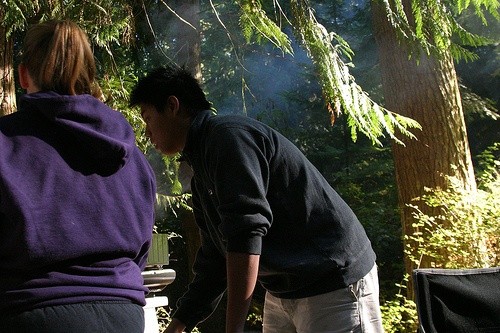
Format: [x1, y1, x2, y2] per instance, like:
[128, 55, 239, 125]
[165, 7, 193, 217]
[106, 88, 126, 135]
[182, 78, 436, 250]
[413, 268, 500, 333]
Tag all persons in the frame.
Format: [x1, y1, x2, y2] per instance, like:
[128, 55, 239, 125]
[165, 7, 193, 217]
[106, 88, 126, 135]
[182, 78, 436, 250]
[0, 20, 157, 333]
[128, 67, 384, 333]
